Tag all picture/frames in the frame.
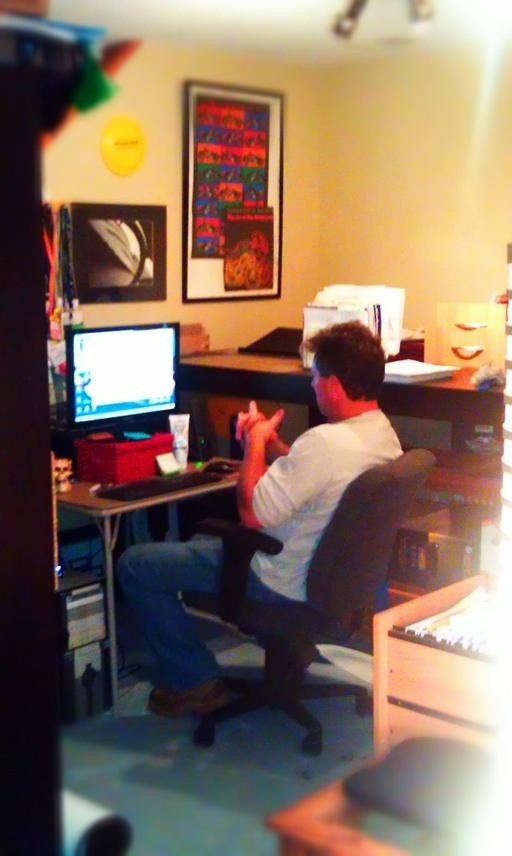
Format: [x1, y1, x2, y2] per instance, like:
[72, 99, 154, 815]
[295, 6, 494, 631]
[70, 201, 168, 301]
[182, 79, 284, 302]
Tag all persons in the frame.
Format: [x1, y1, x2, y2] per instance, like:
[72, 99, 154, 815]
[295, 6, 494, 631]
[115, 314, 405, 724]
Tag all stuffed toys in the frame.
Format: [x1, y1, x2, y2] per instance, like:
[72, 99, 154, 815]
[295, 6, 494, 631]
[54, 457, 75, 495]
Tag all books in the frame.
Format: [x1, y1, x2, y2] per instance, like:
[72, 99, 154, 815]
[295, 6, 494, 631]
[297, 282, 462, 385]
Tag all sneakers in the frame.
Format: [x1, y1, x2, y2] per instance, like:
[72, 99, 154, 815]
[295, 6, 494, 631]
[147, 677, 230, 718]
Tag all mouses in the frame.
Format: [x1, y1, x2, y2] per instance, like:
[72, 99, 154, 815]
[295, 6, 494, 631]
[205, 462, 233, 472]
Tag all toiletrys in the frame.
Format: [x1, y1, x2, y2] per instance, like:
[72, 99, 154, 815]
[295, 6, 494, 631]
[167, 413, 191, 469]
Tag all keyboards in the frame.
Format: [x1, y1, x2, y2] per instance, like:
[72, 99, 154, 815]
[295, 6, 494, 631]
[99, 472, 222, 501]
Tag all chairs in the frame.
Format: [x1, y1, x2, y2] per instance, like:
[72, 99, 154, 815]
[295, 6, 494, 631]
[178, 449, 437, 757]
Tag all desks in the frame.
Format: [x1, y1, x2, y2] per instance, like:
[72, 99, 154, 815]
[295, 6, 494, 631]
[56, 457, 270, 721]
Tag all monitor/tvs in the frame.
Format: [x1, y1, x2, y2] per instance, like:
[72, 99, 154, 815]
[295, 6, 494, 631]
[64, 323, 179, 441]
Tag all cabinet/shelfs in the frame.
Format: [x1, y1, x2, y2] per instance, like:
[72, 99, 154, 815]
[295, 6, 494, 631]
[437, 302, 506, 375]
[180, 359, 504, 639]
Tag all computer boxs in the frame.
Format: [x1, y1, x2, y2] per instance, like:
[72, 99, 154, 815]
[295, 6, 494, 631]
[57, 573, 113, 726]
